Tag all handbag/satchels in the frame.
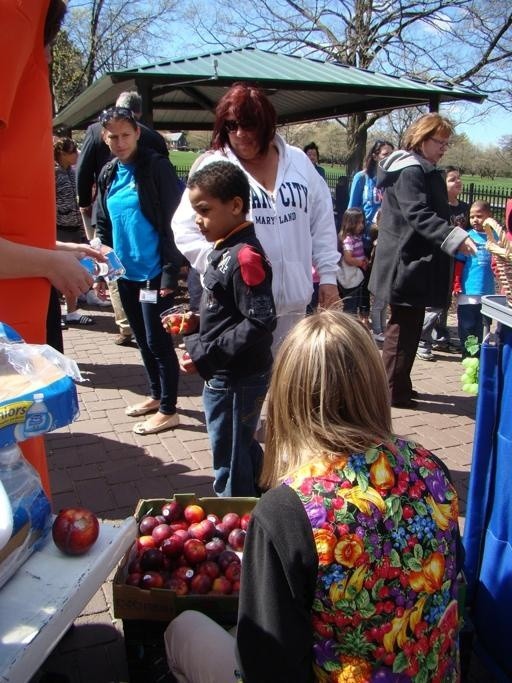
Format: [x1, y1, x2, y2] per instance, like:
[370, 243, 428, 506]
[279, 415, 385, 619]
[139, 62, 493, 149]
[336, 254, 364, 289]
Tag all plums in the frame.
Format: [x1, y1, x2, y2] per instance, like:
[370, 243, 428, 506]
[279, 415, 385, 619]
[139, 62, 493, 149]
[124, 501, 251, 596]
[168, 316, 190, 333]
[184, 352, 191, 370]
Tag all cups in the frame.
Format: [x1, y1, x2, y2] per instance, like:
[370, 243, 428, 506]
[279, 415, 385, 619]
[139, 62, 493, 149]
[159, 303, 190, 338]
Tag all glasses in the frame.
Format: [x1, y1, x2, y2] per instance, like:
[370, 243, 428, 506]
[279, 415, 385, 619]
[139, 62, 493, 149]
[431, 137, 448, 147]
[226, 121, 255, 132]
[99, 108, 136, 126]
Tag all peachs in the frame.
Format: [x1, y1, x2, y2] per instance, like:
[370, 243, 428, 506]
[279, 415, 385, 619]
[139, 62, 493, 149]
[370, 450, 395, 489]
[407, 551, 446, 592]
[312, 528, 365, 569]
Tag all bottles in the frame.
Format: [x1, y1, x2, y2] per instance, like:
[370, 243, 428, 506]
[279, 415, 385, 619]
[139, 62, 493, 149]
[79, 254, 109, 279]
[0, 444, 31, 497]
[89, 236, 126, 283]
[25, 392, 52, 437]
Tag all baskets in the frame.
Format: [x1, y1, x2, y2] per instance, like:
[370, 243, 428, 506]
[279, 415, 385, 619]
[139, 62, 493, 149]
[483, 218, 512, 309]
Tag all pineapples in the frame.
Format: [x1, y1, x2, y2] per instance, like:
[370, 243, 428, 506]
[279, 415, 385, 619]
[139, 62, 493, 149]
[332, 624, 379, 683]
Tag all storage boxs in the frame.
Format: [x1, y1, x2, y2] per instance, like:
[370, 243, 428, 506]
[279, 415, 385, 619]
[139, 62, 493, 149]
[111, 491, 262, 681]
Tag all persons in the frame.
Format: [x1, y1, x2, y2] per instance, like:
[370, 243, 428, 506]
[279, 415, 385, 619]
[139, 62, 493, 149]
[170, 80, 339, 359]
[0, 0, 109, 501]
[90, 106, 182, 435]
[53, 136, 95, 330]
[164, 306, 467, 683]
[76, 90, 169, 345]
[161, 161, 280, 497]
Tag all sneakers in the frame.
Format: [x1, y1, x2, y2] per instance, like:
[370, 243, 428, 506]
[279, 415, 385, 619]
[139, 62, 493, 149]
[416, 352, 435, 361]
[431, 342, 461, 354]
[79, 290, 112, 306]
[115, 335, 131, 345]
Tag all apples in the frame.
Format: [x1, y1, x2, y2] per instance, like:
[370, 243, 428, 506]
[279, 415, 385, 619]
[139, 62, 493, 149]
[51, 508, 98, 553]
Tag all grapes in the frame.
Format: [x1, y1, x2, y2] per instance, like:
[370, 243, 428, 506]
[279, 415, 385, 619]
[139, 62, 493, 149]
[461, 357, 479, 394]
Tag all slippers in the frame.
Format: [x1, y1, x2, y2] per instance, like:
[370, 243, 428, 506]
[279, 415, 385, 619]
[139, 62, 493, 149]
[67, 315, 95, 325]
[61, 318, 67, 330]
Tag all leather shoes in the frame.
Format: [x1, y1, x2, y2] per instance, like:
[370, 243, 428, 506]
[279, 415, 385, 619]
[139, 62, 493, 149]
[133, 413, 179, 434]
[391, 400, 417, 408]
[125, 403, 160, 415]
[411, 390, 417, 397]
[373, 333, 385, 341]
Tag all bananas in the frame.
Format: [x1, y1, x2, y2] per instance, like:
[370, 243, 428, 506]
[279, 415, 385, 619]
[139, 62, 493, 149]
[425, 494, 452, 530]
[328, 563, 366, 602]
[382, 596, 427, 652]
[336, 485, 387, 515]
